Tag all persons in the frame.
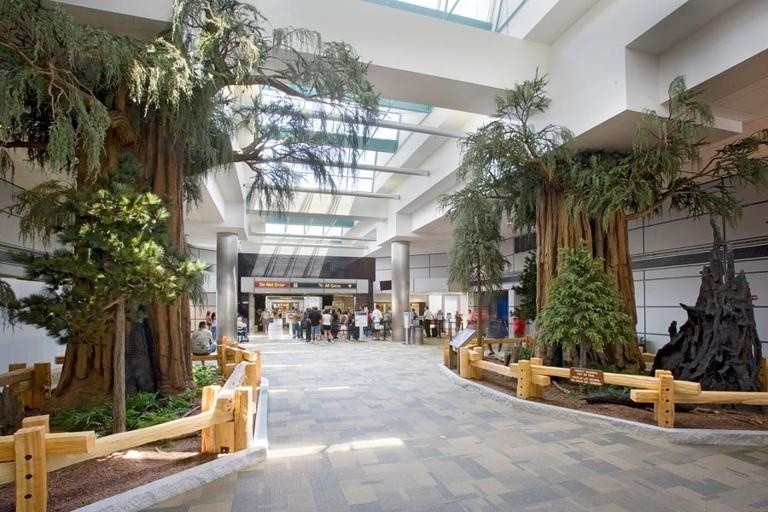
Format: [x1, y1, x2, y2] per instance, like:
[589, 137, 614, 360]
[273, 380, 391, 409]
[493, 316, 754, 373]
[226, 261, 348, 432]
[259, 307, 282, 335]
[466, 310, 473, 325]
[242, 314, 249, 342]
[455, 311, 461, 332]
[513, 317, 526, 347]
[291, 304, 447, 345]
[486, 313, 505, 355]
[192, 311, 217, 366]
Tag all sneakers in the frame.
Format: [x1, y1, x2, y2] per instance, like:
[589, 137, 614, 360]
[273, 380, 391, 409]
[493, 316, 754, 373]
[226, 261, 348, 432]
[371, 336, 380, 341]
[292, 334, 359, 344]
